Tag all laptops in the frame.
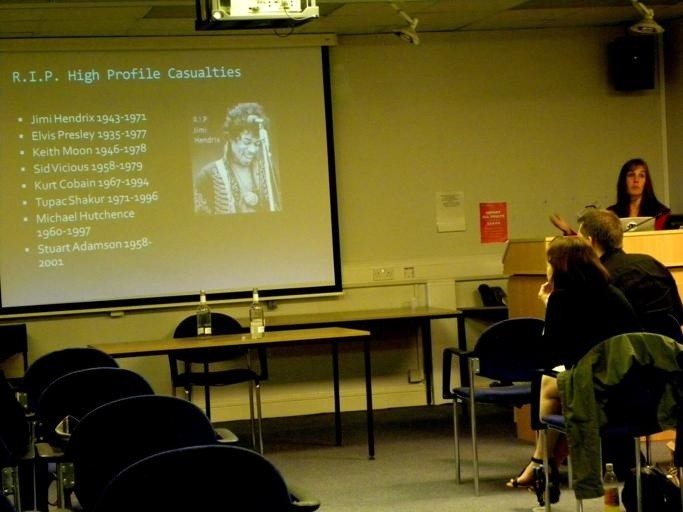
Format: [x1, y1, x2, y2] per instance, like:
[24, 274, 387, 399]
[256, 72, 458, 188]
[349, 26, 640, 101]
[616, 216, 656, 233]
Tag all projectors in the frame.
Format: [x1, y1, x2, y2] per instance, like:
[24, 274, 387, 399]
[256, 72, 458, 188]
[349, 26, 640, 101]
[200, 0, 320, 28]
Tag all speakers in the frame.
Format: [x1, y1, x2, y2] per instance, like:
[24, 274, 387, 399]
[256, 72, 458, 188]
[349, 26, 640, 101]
[606, 21, 655, 92]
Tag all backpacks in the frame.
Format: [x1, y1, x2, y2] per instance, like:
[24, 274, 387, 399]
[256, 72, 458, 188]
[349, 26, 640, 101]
[621, 464, 682, 511]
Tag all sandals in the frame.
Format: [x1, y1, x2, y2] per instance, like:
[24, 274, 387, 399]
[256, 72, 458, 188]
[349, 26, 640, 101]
[505, 457, 554, 489]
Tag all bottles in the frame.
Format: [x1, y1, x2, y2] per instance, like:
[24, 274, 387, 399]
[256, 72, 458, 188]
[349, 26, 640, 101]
[602, 464, 620, 512]
[249, 288, 265, 340]
[197, 290, 211, 339]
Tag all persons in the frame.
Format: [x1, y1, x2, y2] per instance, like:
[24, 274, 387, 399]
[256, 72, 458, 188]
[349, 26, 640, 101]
[548, 156, 672, 237]
[576, 207, 681, 337]
[504, 234, 644, 491]
[194, 101, 276, 213]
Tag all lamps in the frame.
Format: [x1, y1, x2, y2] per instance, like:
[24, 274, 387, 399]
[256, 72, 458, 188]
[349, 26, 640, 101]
[384, 2, 423, 48]
[628, 0, 663, 36]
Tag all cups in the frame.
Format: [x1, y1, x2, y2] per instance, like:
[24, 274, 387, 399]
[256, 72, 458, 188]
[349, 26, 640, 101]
[402, 267, 414, 279]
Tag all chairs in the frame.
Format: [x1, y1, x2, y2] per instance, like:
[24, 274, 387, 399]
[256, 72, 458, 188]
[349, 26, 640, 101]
[442, 316, 546, 496]
[1, 321, 33, 510]
[527, 331, 682, 510]
[103, 445, 291, 511]
[69, 394, 216, 508]
[169, 311, 264, 455]
[25, 344, 153, 509]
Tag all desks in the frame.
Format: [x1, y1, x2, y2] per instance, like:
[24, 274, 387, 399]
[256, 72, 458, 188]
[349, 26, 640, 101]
[86, 325, 378, 461]
[233, 301, 469, 445]
[455, 306, 510, 429]
